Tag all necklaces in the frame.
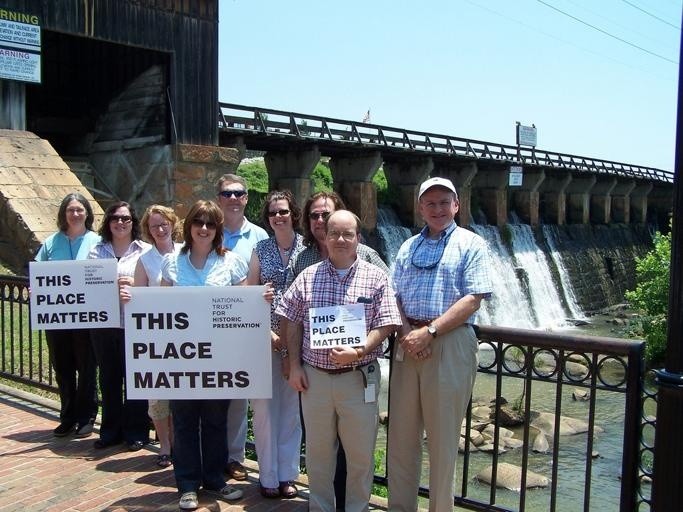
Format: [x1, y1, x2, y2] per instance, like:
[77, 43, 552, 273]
[273, 239, 291, 255]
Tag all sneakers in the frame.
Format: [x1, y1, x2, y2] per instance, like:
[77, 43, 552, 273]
[204, 484, 243, 500]
[260, 483, 280, 498]
[227, 461, 248, 480]
[179, 491, 199, 509]
[280, 481, 298, 498]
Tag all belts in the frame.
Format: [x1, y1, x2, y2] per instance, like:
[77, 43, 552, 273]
[316, 364, 370, 387]
[407, 318, 431, 327]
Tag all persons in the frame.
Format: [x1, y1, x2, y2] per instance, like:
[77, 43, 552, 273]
[247, 186, 311, 496]
[84, 200, 151, 450]
[383, 171, 493, 511]
[133, 203, 188, 468]
[271, 207, 404, 511]
[120, 200, 275, 508]
[38, 191, 97, 439]
[205, 174, 267, 480]
[280, 188, 393, 512]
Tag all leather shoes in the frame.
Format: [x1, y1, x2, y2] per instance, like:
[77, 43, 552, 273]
[128, 440, 144, 451]
[75, 424, 94, 438]
[94, 439, 106, 449]
[54, 423, 76, 436]
[158, 454, 172, 467]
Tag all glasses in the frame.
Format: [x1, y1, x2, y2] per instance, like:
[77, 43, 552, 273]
[268, 209, 291, 217]
[192, 220, 216, 230]
[412, 262, 435, 270]
[68, 208, 84, 215]
[325, 229, 356, 240]
[151, 220, 169, 230]
[111, 215, 132, 224]
[217, 190, 247, 198]
[309, 211, 331, 221]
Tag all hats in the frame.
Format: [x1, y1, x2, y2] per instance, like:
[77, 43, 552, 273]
[417, 176, 456, 201]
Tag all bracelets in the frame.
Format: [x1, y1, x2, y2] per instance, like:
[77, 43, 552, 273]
[279, 345, 288, 358]
[354, 347, 365, 361]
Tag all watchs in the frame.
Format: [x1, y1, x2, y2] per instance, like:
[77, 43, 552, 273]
[425, 323, 437, 339]
